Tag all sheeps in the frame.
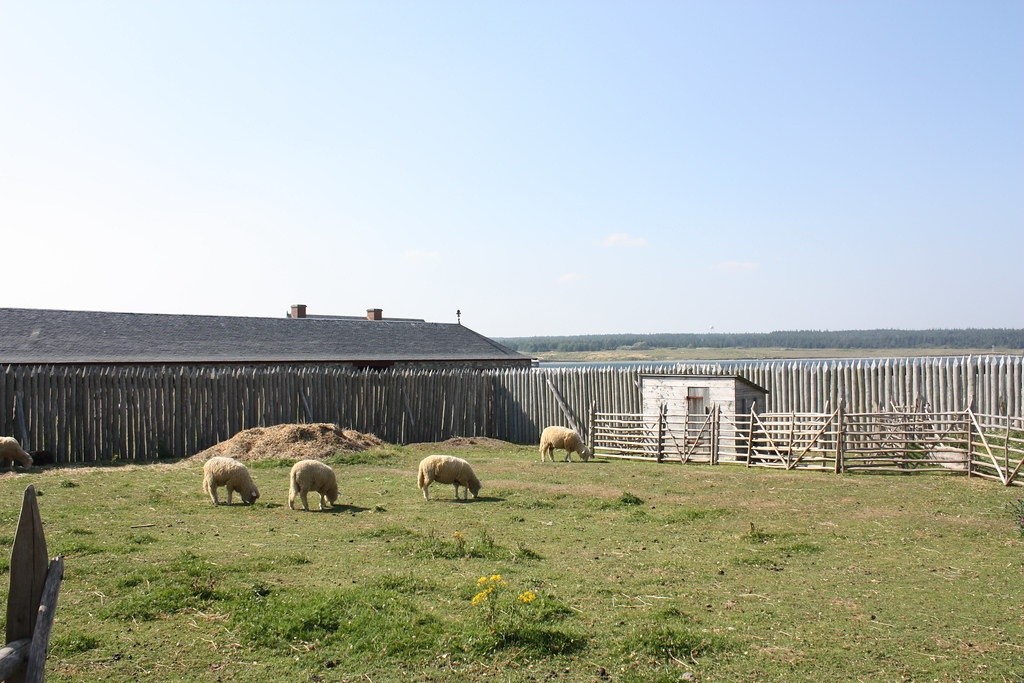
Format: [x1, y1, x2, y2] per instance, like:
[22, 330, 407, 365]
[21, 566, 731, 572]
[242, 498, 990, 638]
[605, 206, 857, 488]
[289, 460, 339, 511]
[0, 436, 33, 471]
[203, 456, 260, 505]
[418, 455, 482, 501]
[540, 426, 592, 463]
